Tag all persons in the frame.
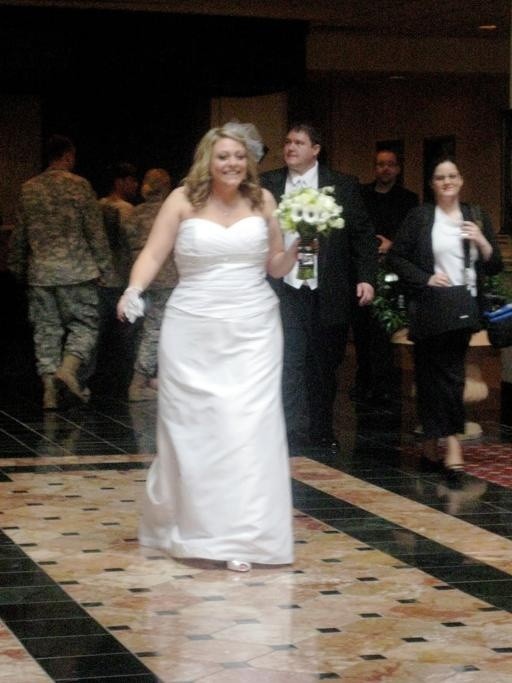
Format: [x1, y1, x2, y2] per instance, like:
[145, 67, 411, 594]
[257, 120, 378, 454]
[7, 133, 114, 413]
[97, 161, 138, 395]
[118, 166, 180, 401]
[114, 115, 321, 574]
[357, 140, 423, 454]
[388, 153, 505, 472]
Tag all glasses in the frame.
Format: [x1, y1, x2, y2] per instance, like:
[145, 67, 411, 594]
[375, 160, 399, 169]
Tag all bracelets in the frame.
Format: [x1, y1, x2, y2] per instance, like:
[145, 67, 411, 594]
[121, 284, 146, 296]
[477, 240, 491, 248]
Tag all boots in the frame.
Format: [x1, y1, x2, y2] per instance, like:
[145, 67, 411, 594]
[52, 351, 92, 404]
[128, 370, 160, 403]
[40, 371, 60, 412]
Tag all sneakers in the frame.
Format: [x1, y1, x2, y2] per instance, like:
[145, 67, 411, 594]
[442, 460, 467, 486]
[312, 435, 342, 452]
[416, 453, 445, 472]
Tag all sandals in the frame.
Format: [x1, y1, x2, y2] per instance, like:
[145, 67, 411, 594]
[225, 559, 253, 573]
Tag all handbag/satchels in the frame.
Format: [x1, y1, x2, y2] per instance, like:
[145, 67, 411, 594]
[407, 284, 476, 342]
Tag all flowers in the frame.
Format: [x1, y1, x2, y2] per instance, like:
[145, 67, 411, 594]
[270, 184, 349, 238]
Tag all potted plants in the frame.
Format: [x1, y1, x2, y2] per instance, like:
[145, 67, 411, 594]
[369, 266, 512, 441]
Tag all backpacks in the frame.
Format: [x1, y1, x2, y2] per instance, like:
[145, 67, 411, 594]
[97, 201, 121, 251]
